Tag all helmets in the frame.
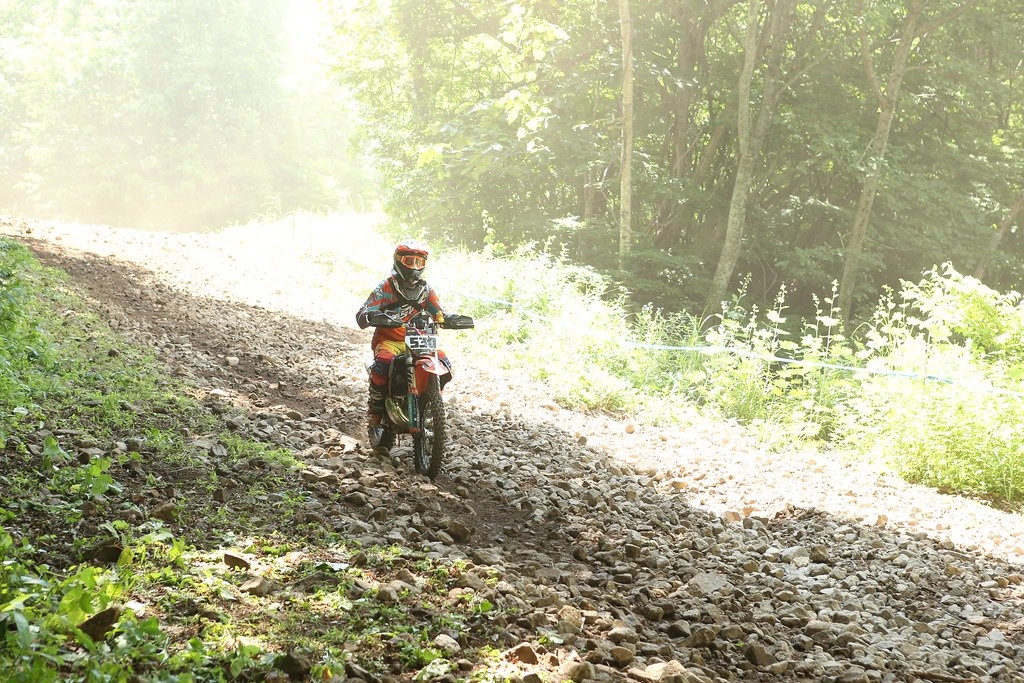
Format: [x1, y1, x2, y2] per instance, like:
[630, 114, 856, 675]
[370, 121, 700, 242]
[393, 239, 429, 283]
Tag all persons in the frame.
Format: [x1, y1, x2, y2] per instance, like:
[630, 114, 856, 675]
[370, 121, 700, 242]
[356, 240, 451, 427]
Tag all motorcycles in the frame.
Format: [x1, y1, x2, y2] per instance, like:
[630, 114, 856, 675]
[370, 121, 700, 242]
[364, 309, 474, 480]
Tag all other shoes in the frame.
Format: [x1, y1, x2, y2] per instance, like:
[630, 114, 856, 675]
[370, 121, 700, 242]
[367, 412, 382, 426]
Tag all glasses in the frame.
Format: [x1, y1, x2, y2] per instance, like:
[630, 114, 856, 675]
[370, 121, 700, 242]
[402, 256, 425, 268]
